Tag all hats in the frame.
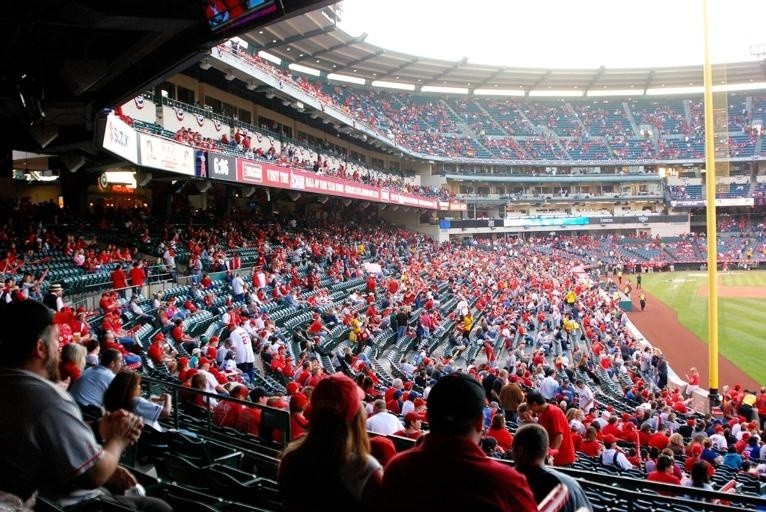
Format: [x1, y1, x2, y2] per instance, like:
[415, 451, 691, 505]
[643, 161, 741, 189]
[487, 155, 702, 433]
[100, 289, 119, 332]
[634, 374, 686, 414]
[225, 300, 287, 356]
[682, 410, 760, 466]
[0, 295, 72, 330]
[429, 371, 492, 420]
[47, 282, 62, 293]
[309, 374, 368, 421]
[393, 381, 430, 426]
[234, 383, 308, 410]
[180, 334, 221, 377]
[603, 413, 640, 444]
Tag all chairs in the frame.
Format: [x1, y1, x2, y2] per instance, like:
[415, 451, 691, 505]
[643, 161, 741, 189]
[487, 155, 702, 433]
[0, 80, 766, 512]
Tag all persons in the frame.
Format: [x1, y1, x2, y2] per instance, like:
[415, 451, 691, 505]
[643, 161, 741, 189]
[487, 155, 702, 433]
[638, 290, 647, 312]
[625, 280, 633, 297]
[607, 294, 766, 511]
[617, 270, 623, 284]
[0, 97, 766, 512]
[218, 61, 362, 98]
[608, 269, 614, 279]
[607, 91, 766, 269]
[636, 274, 642, 289]
